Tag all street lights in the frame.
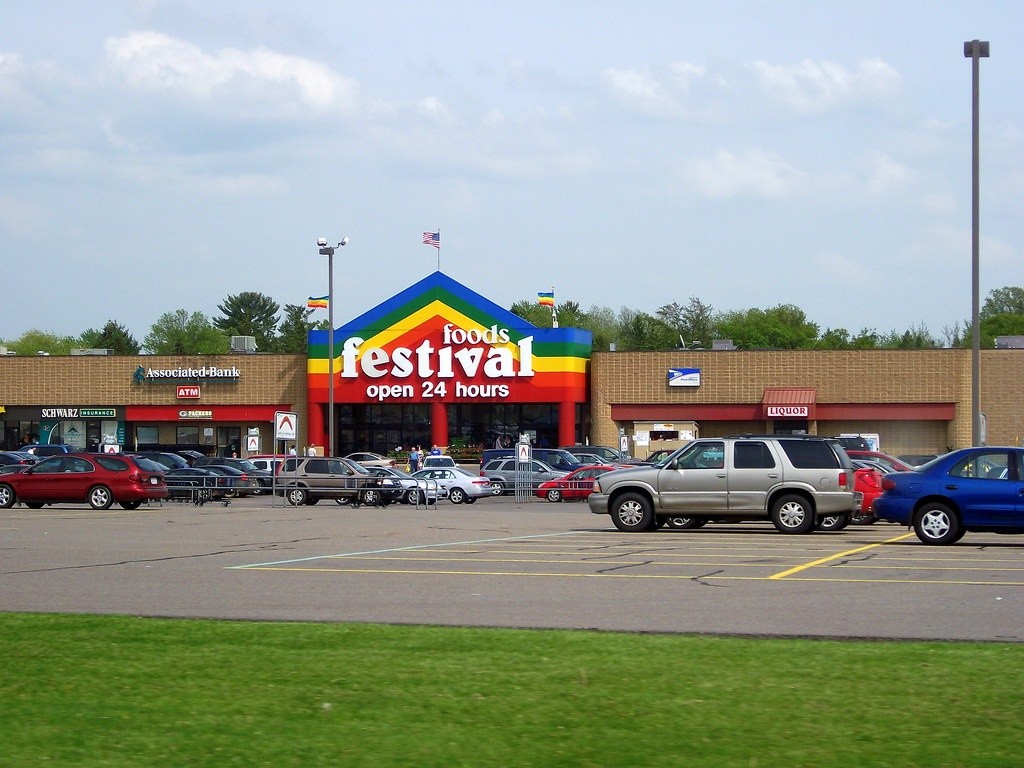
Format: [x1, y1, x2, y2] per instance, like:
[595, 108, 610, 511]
[316, 233, 349, 456]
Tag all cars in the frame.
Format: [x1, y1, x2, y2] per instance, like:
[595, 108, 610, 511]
[338, 451, 399, 471]
[411, 467, 492, 505]
[535, 464, 617, 503]
[851, 461, 883, 527]
[0, 451, 39, 469]
[872, 443, 1023, 546]
[574, 453, 637, 471]
[202, 464, 259, 501]
[335, 466, 451, 507]
[158, 467, 235, 503]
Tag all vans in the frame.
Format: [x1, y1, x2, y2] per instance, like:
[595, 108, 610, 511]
[239, 457, 287, 474]
[481, 448, 595, 474]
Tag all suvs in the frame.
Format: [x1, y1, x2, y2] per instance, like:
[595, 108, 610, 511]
[587, 434, 864, 535]
[480, 456, 575, 498]
[420, 455, 460, 470]
[274, 457, 403, 508]
[131, 450, 188, 469]
[0, 452, 170, 513]
[179, 449, 276, 496]
[563, 445, 645, 464]
[18, 444, 79, 462]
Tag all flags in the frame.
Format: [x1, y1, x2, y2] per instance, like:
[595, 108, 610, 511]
[422, 231, 440, 248]
[538, 292, 554, 306]
[308, 296, 328, 308]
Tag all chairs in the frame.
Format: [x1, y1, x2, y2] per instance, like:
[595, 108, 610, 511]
[65, 460, 76, 472]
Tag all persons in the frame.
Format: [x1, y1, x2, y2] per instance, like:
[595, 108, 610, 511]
[288, 445, 296, 455]
[488, 433, 550, 449]
[416, 446, 424, 470]
[431, 445, 441, 454]
[307, 443, 317, 456]
[20, 434, 29, 450]
[407, 447, 420, 473]
